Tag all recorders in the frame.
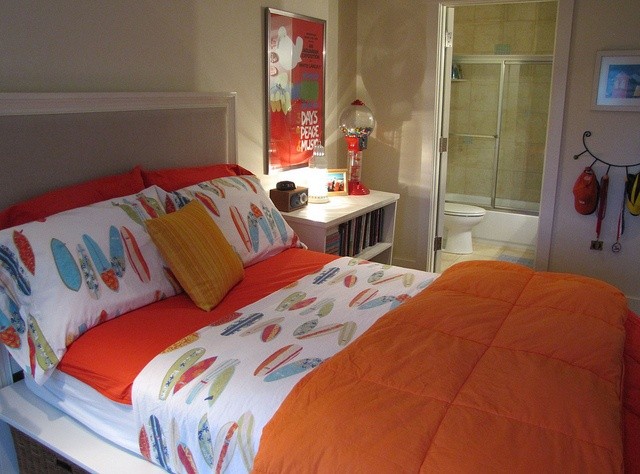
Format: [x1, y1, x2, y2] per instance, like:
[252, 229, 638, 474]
[270, 186, 308, 212]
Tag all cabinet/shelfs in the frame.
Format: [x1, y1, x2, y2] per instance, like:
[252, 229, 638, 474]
[452, 77, 466, 82]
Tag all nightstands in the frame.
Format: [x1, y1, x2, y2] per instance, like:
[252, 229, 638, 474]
[279, 187, 400, 266]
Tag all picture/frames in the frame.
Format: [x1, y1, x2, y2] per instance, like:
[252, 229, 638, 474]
[265, 6, 327, 175]
[589, 50, 640, 111]
[326, 168, 350, 196]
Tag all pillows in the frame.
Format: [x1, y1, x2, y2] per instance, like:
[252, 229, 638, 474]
[167, 175, 309, 268]
[0, 165, 147, 229]
[144, 199, 243, 313]
[0, 184, 182, 385]
[140, 163, 254, 193]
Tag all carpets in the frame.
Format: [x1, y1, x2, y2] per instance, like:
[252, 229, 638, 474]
[496, 253, 533, 270]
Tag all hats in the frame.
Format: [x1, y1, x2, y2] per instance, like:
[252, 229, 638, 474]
[573, 168, 600, 215]
[627, 173, 640, 216]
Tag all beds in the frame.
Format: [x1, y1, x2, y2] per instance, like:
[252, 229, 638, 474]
[1, 91, 627, 474]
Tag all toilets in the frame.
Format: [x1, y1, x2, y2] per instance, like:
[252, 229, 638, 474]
[442, 202, 486, 255]
